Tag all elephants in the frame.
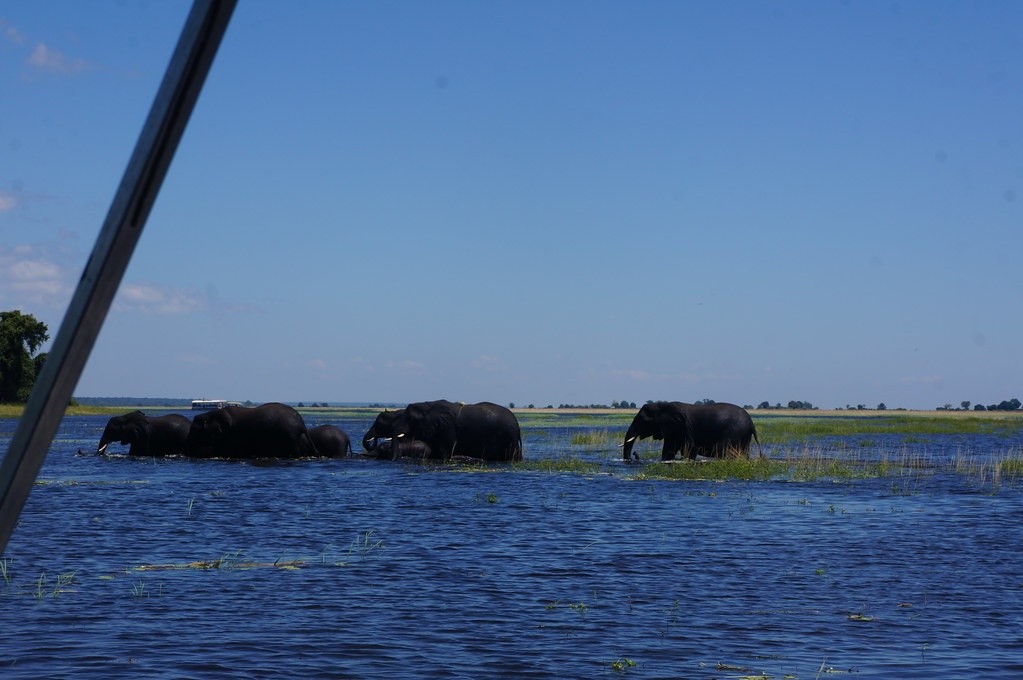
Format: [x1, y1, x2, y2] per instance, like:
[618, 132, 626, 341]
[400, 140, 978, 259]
[362, 399, 524, 463]
[618, 401, 763, 463]
[77, 402, 352, 460]
